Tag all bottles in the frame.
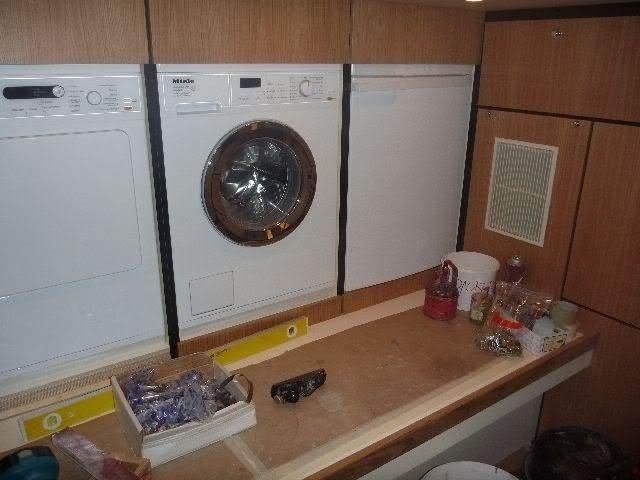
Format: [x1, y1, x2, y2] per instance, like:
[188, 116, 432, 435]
[550, 301, 578, 327]
[504, 254, 524, 282]
[469, 292, 492, 326]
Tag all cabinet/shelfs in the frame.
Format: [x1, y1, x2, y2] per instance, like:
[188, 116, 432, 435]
[2, 283, 601, 479]
[456, 5, 640, 478]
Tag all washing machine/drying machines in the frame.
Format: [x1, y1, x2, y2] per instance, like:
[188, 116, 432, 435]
[151, 62, 344, 343]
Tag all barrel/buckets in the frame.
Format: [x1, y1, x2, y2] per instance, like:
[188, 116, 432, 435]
[440, 251, 501, 312]
[420, 461, 520, 480]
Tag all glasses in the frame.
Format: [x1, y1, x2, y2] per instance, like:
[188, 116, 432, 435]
[271, 368, 326, 403]
